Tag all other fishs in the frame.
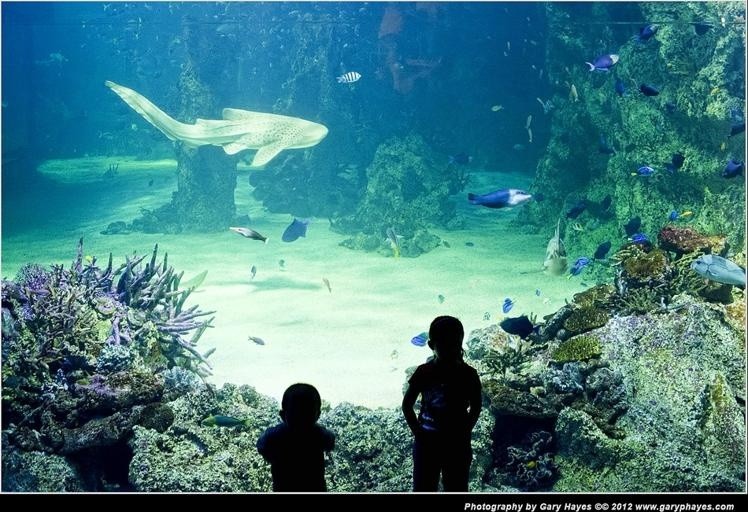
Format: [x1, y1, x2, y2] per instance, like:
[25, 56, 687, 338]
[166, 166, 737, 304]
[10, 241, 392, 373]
[386, 13, 745, 349]
[104, 77, 328, 171]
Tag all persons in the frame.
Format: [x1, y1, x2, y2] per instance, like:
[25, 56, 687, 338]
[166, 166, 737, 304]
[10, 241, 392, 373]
[256, 384, 335, 492]
[402, 316, 482, 492]
